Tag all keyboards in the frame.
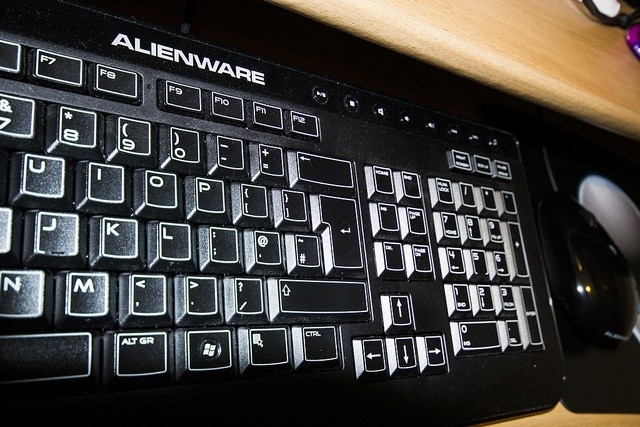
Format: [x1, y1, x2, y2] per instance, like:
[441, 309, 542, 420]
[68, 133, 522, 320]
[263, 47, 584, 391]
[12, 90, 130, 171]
[0, 1, 563, 425]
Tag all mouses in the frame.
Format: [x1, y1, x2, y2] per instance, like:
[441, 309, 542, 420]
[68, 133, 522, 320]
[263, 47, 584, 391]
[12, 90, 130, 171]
[536, 192, 637, 351]
